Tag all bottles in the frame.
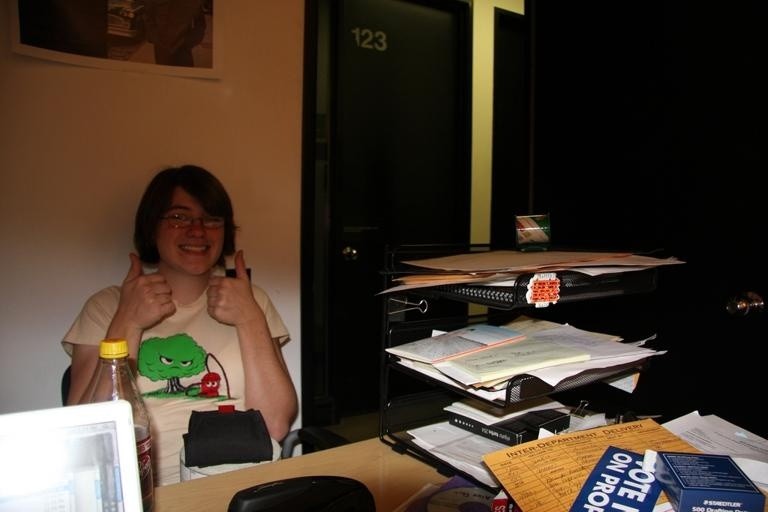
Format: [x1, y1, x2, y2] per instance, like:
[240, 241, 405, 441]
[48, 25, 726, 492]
[73, 335, 158, 512]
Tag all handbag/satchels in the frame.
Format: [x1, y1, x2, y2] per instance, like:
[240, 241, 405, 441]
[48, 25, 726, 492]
[183, 408, 273, 468]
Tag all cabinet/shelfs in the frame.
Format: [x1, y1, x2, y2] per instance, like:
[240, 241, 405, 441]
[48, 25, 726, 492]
[367, 241, 672, 493]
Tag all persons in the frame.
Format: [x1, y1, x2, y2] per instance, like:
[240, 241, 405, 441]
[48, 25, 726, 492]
[62, 163, 300, 483]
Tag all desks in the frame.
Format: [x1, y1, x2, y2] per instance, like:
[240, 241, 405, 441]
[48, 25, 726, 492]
[137, 414, 767, 512]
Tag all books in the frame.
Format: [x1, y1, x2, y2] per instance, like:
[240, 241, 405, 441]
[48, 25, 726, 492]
[384, 323, 592, 383]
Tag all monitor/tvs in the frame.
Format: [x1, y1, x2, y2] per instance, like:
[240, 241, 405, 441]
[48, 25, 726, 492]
[3, 397, 144, 511]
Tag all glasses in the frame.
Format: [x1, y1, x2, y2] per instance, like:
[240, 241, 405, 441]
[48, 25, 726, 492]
[161, 213, 225, 230]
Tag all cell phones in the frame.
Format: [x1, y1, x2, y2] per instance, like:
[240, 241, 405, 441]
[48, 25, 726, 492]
[223, 268, 252, 280]
